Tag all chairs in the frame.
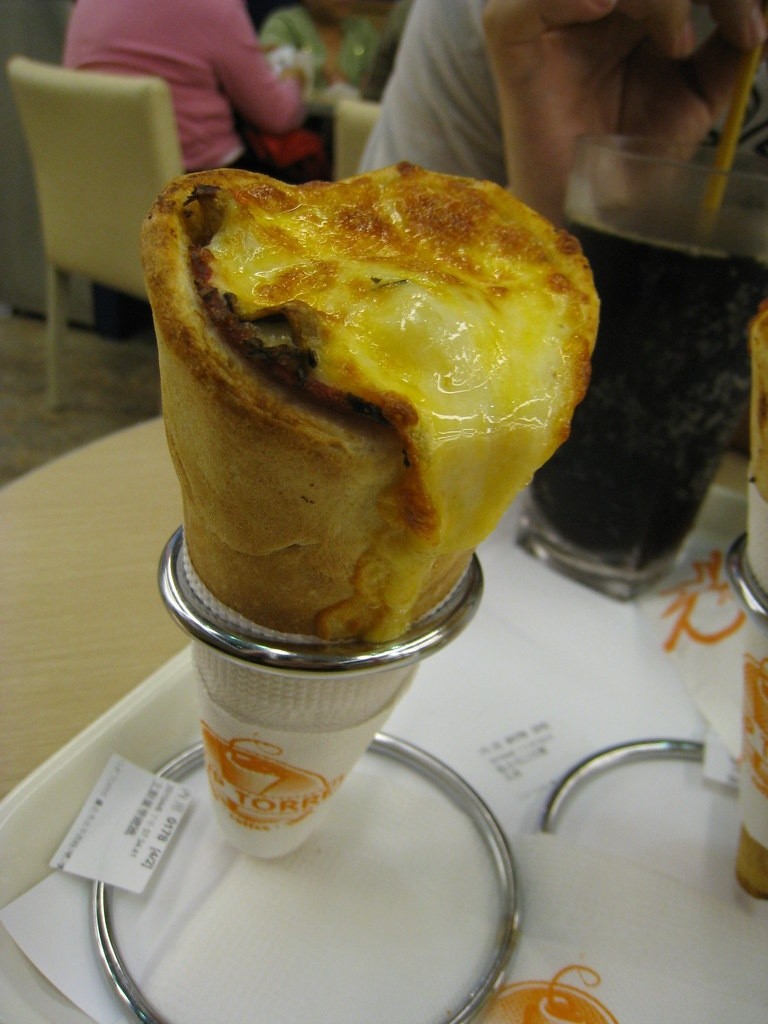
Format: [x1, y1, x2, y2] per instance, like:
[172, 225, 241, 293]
[332, 95, 381, 179]
[7, 56, 185, 412]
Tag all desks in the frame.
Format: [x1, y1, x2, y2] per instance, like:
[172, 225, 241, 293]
[0, 414, 768, 1024]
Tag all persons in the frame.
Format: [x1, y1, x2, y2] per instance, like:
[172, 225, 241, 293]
[63, 0, 768, 462]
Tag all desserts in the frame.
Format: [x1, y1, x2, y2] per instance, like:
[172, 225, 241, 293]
[138, 164, 600, 646]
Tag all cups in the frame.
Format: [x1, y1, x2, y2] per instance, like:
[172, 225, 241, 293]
[512, 134, 768, 598]
